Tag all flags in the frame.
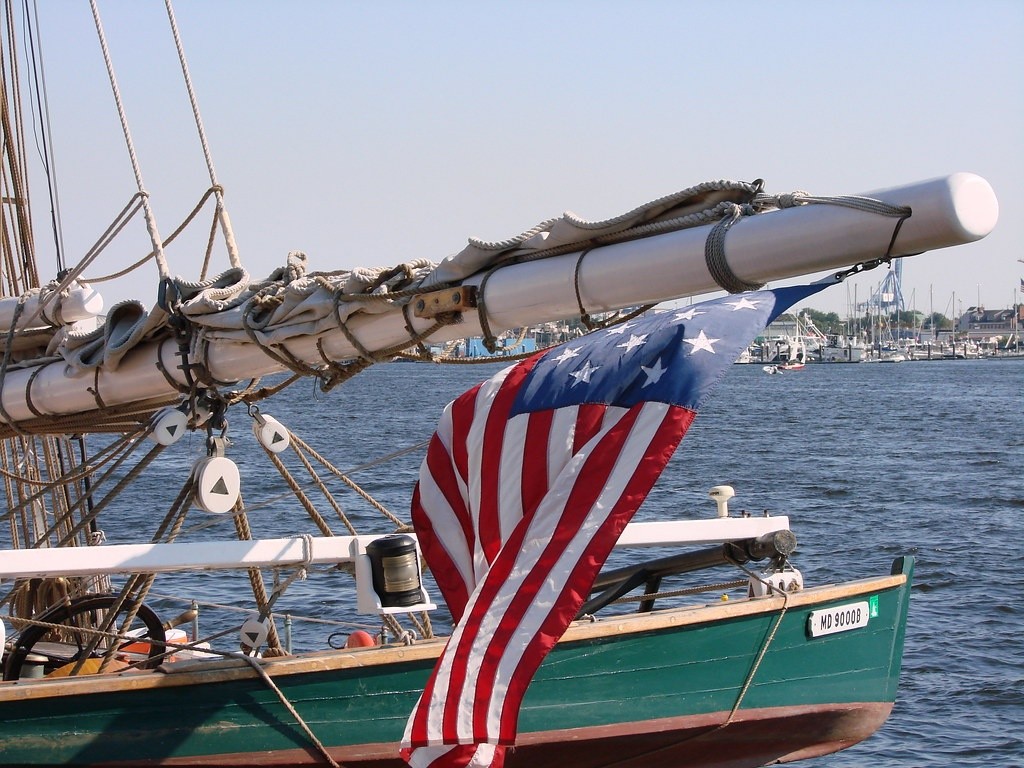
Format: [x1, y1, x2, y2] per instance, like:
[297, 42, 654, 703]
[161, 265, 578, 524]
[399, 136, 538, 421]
[401, 273, 839, 768]
[1020, 279, 1024, 293]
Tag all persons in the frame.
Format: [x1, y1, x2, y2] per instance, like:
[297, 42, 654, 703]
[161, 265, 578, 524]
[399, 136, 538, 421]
[770, 365, 777, 370]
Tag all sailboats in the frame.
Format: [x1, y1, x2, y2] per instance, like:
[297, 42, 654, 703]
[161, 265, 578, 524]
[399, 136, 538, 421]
[0, 3, 998, 768]
[845, 280, 985, 362]
[762, 366, 783, 375]
[775, 309, 807, 370]
